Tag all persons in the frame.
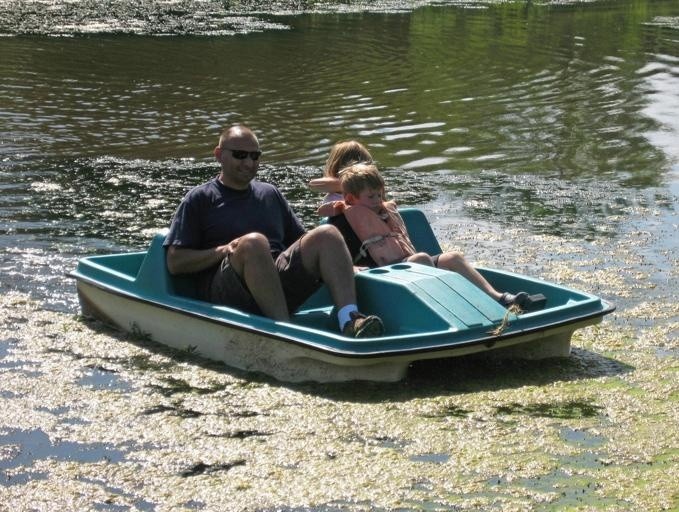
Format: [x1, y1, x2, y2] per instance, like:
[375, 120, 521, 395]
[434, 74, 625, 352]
[309, 141, 397, 215]
[327, 162, 531, 311]
[162, 125, 387, 338]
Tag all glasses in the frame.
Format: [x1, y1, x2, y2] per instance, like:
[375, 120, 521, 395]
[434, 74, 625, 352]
[219, 146, 260, 161]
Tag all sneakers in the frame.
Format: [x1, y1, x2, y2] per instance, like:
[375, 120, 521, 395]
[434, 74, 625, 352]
[342, 310, 385, 337]
[501, 292, 528, 313]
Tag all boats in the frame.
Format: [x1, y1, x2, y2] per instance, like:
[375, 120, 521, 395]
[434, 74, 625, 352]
[60, 205, 617, 387]
